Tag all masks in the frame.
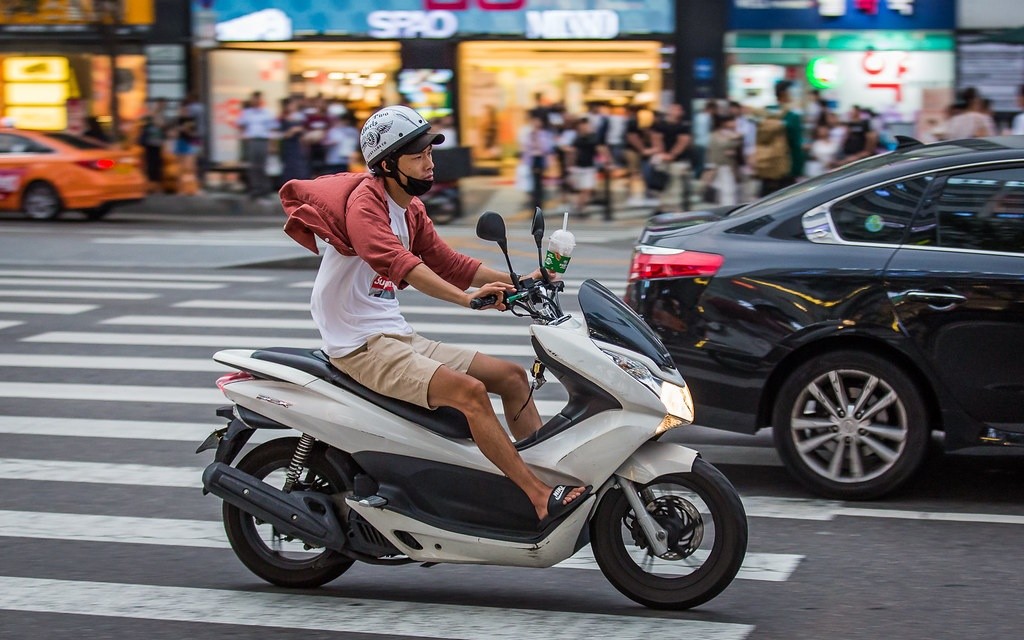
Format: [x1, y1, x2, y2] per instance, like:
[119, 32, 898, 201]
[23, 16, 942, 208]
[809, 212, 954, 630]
[393, 164, 435, 196]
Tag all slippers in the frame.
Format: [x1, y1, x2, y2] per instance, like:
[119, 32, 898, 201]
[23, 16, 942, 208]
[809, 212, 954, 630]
[535, 485, 594, 533]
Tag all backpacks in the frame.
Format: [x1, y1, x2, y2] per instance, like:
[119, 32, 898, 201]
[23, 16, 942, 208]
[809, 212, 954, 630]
[750, 106, 793, 181]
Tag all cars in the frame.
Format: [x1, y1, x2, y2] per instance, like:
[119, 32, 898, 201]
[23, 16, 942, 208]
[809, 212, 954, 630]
[623, 134, 1024, 502]
[0, 121, 159, 224]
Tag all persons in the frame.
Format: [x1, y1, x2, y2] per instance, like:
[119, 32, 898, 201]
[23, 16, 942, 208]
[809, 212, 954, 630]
[1010, 84, 1024, 135]
[77, 98, 206, 196]
[236, 91, 467, 218]
[755, 80, 807, 200]
[309, 105, 594, 533]
[515, 89, 899, 222]
[924, 86, 997, 146]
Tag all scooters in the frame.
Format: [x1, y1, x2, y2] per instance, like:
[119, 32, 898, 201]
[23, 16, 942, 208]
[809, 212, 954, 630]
[194, 206, 750, 612]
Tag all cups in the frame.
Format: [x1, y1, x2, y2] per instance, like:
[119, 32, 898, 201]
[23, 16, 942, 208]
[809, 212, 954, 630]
[543, 230, 576, 294]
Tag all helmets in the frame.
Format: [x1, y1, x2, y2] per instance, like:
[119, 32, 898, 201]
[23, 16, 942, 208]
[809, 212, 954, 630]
[360, 105, 445, 168]
